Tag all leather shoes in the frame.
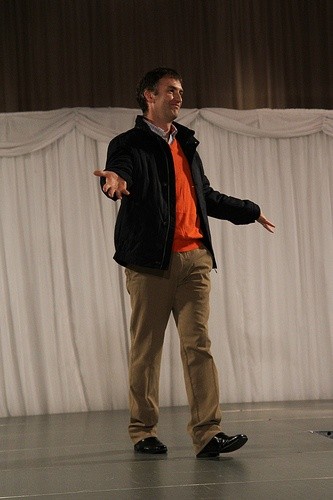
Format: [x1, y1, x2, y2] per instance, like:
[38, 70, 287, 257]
[196, 432, 248, 458]
[134, 437, 167, 453]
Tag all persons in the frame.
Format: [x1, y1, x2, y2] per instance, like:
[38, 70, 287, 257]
[93, 67, 277, 458]
